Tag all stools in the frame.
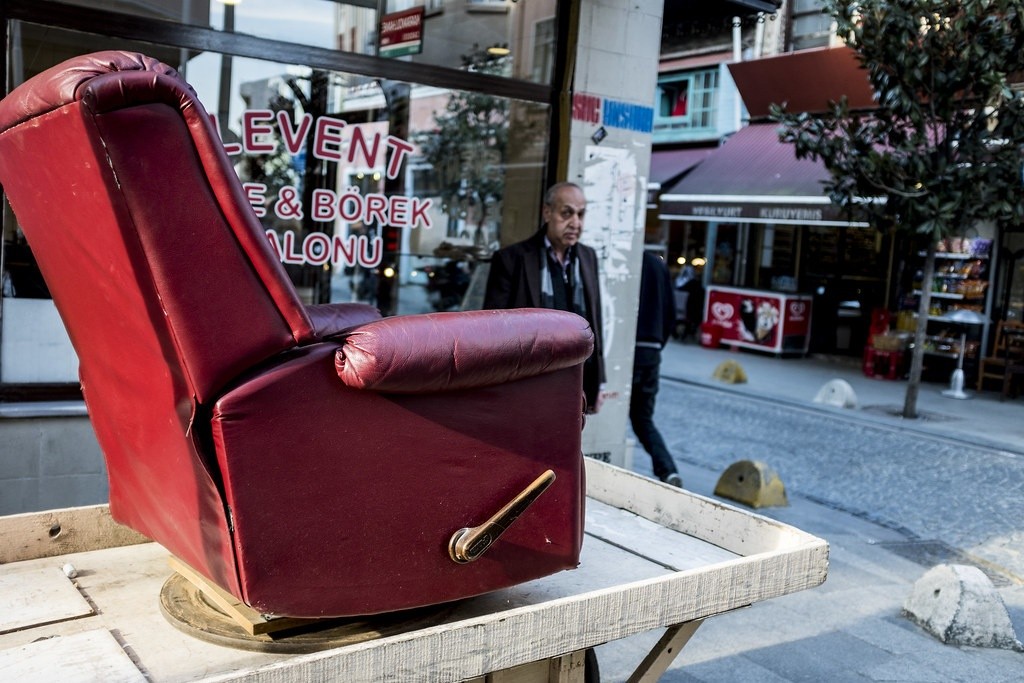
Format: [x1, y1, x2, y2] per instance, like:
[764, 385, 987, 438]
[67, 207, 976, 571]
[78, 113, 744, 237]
[862, 343, 904, 382]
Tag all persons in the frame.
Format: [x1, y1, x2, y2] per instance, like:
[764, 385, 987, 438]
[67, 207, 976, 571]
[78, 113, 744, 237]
[482, 183, 608, 429]
[629, 248, 706, 488]
[425, 249, 470, 315]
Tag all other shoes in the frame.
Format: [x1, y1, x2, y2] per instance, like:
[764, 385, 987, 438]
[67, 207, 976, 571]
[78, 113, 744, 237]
[660, 470, 683, 488]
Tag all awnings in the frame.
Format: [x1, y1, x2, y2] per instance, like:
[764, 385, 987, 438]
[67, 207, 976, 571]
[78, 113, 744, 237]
[647, 116, 950, 228]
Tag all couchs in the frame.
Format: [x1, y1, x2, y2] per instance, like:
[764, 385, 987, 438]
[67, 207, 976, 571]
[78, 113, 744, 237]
[0, 50, 594, 621]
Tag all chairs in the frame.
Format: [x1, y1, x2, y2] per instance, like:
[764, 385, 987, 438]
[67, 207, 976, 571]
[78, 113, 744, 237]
[978, 319, 1024, 400]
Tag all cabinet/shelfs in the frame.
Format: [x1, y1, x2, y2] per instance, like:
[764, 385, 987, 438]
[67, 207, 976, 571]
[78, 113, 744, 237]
[907, 236, 993, 392]
